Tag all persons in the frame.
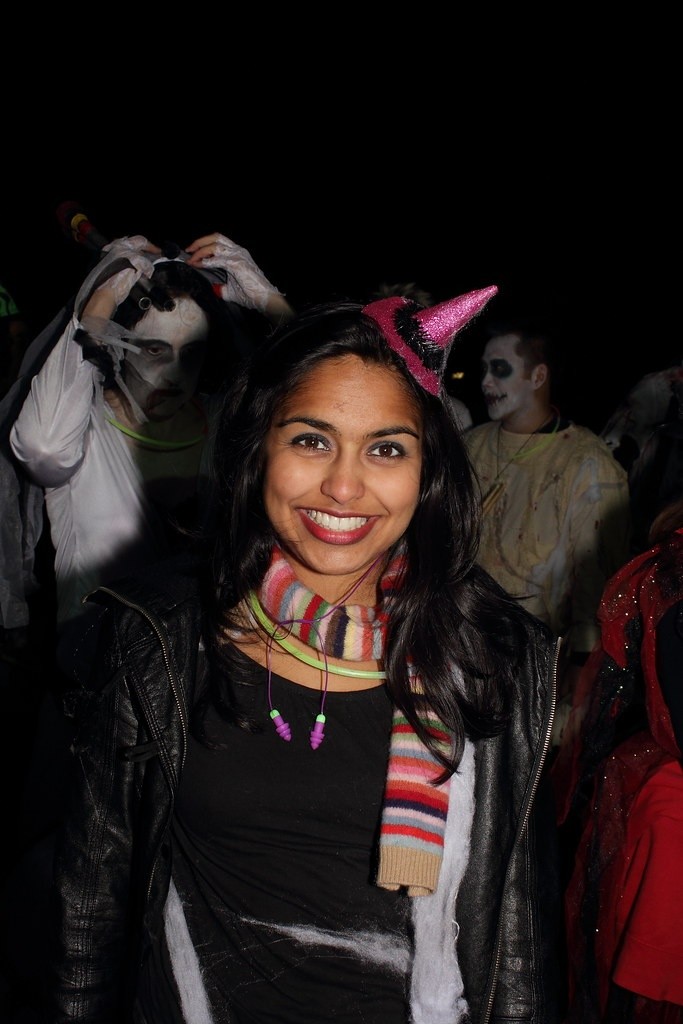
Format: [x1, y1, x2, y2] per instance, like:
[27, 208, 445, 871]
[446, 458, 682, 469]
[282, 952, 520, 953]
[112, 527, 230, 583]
[0, 192, 683, 1024]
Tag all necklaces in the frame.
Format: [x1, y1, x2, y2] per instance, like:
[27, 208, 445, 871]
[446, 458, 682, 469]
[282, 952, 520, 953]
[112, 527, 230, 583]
[245, 585, 388, 681]
[100, 405, 206, 448]
[485, 403, 561, 459]
[489, 412, 555, 487]
